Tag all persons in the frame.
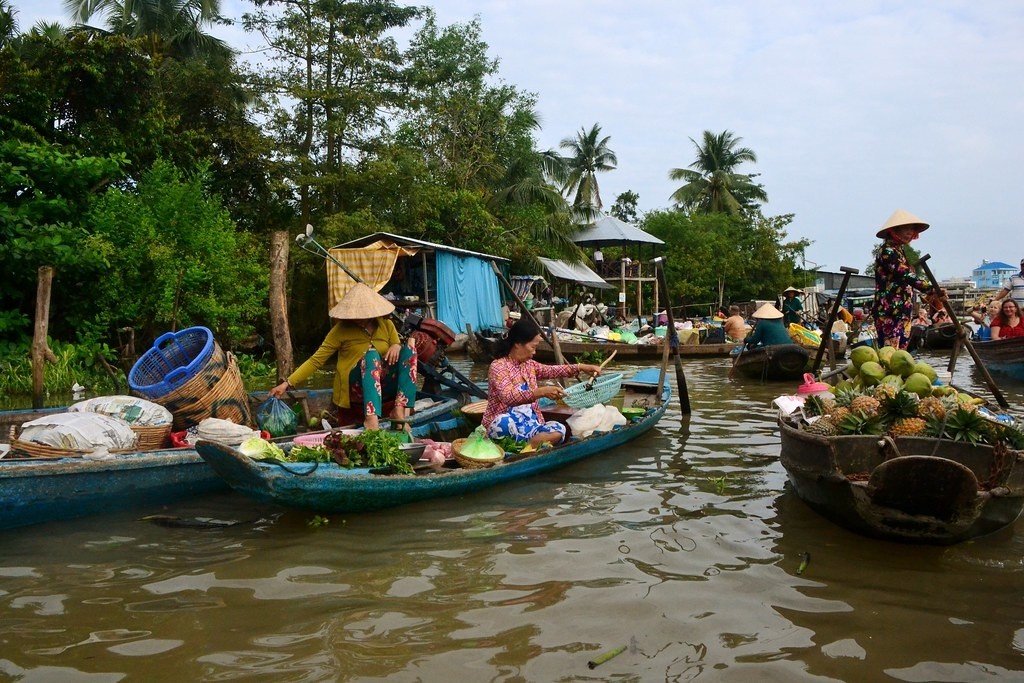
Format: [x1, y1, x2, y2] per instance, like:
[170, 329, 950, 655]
[917, 292, 943, 317]
[970, 300, 1002, 340]
[912, 309, 933, 326]
[744, 301, 795, 346]
[782, 286, 804, 326]
[871, 208, 949, 354]
[932, 307, 953, 324]
[836, 306, 853, 324]
[476, 320, 604, 449]
[988, 299, 1024, 340]
[994, 258, 1024, 311]
[267, 283, 419, 435]
[722, 305, 746, 342]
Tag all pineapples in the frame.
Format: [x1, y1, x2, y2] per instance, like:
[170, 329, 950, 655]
[804, 375, 1024, 447]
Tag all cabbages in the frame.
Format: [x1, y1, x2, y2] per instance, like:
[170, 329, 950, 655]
[238, 436, 286, 461]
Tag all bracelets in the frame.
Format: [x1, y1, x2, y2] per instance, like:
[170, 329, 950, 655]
[286, 379, 292, 388]
[981, 321, 985, 324]
[577, 363, 582, 372]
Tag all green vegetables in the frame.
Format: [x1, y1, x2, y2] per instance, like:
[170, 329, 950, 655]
[500, 437, 527, 454]
[290, 427, 416, 475]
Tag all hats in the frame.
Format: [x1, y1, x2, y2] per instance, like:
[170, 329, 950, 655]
[782, 286, 800, 296]
[329, 283, 396, 319]
[752, 302, 784, 319]
[876, 209, 929, 239]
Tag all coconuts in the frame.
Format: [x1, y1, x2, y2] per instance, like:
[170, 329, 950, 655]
[835, 346, 973, 402]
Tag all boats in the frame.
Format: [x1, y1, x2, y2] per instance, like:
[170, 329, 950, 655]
[0, 224, 489, 530]
[474, 292, 1024, 382]
[195, 253, 690, 510]
[777, 253, 1024, 542]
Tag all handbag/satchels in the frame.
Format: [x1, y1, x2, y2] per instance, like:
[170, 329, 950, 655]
[572, 303, 586, 319]
[256, 395, 298, 439]
[461, 424, 502, 461]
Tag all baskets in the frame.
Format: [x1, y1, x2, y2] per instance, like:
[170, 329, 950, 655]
[558, 374, 624, 409]
[452, 438, 505, 468]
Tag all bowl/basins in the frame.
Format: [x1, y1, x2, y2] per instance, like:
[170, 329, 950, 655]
[623, 407, 645, 419]
[399, 442, 428, 466]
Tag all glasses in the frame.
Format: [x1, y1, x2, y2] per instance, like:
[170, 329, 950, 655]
[1003, 306, 1017, 310]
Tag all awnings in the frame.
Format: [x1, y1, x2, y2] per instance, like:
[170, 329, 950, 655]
[536, 256, 618, 288]
